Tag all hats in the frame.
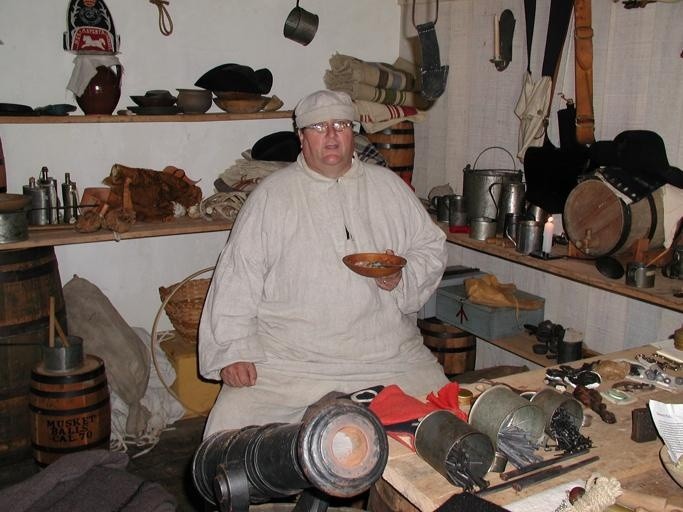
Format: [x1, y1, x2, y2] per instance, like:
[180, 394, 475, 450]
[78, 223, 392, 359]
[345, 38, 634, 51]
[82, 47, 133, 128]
[603, 130, 683, 189]
[294, 89, 356, 128]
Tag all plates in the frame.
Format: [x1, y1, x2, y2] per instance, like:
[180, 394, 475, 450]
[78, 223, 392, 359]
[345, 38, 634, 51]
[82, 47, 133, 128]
[127, 106, 183, 115]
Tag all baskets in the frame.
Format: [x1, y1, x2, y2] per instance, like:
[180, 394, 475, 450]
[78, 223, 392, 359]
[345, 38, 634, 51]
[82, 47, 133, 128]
[159, 277, 212, 344]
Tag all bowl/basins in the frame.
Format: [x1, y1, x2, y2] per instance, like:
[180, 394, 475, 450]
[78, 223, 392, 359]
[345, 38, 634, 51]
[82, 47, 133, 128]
[211, 97, 271, 114]
[213, 90, 262, 99]
[129, 95, 177, 106]
[0, 193, 33, 212]
[342, 253, 407, 278]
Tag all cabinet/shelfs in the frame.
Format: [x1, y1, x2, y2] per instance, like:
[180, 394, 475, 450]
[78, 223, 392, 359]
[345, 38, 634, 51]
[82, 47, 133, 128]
[430, 214, 682, 367]
[0, 109, 296, 251]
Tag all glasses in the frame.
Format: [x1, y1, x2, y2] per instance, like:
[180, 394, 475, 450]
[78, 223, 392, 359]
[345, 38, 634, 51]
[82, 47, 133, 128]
[299, 120, 354, 133]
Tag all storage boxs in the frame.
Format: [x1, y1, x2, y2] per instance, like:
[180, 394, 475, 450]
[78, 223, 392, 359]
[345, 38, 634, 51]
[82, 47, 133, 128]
[157, 330, 221, 420]
[435, 283, 545, 340]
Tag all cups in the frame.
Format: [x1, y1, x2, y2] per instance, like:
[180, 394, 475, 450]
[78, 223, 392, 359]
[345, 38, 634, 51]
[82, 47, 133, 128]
[533, 336, 583, 364]
[626, 262, 656, 288]
[432, 180, 546, 255]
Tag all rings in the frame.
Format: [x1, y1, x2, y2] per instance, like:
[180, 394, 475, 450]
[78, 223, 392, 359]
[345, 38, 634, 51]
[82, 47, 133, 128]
[383, 279, 388, 284]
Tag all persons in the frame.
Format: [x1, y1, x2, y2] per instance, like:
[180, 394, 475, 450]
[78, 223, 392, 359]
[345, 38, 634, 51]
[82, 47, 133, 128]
[198, 90, 450, 441]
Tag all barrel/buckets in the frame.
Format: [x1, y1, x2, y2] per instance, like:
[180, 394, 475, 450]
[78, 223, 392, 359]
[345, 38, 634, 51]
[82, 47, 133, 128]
[359, 121, 415, 184]
[32, 354, 111, 475]
[421, 317, 476, 376]
[461, 147, 523, 219]
[0, 246, 67, 467]
[562, 179, 664, 256]
